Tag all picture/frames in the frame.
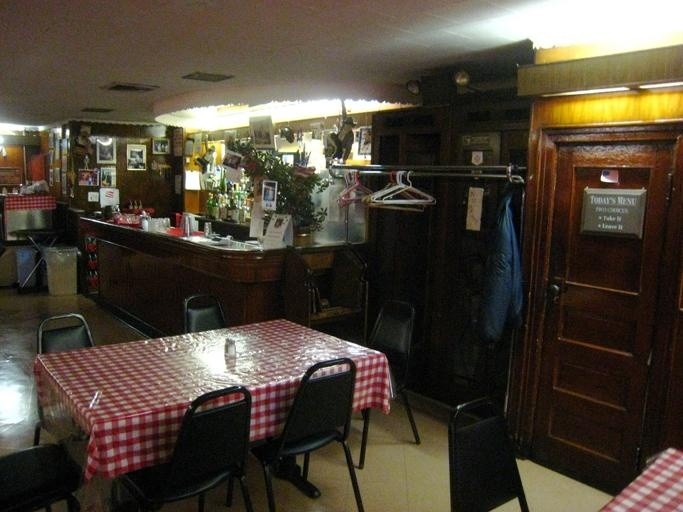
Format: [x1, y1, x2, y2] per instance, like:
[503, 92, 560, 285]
[152, 137, 170, 155]
[77, 132, 117, 187]
[249, 115, 275, 148]
[358, 127, 372, 155]
[126, 143, 146, 171]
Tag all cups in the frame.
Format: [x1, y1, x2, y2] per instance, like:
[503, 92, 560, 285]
[149, 217, 171, 232]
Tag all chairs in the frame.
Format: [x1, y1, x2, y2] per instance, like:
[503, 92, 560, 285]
[349, 299, 423, 468]
[248, 355, 364, 505]
[184, 293, 230, 336]
[113, 384, 256, 508]
[1, 446, 92, 510]
[34, 311, 98, 446]
[449, 399, 531, 512]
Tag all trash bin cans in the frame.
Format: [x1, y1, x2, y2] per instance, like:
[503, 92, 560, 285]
[43, 247, 78, 295]
[17, 249, 36, 288]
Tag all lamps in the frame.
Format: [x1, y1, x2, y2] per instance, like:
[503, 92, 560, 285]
[407, 79, 423, 97]
[454, 70, 482, 93]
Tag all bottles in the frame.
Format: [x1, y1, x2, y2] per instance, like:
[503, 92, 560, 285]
[204, 176, 254, 224]
[141, 216, 149, 232]
[225, 337, 236, 358]
[128, 200, 143, 209]
[85, 234, 100, 291]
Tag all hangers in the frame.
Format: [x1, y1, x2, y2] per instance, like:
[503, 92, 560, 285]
[336, 169, 438, 213]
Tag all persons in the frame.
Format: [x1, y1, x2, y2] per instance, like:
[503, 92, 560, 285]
[154, 143, 168, 152]
[264, 186, 274, 201]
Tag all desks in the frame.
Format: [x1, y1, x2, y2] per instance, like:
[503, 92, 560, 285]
[1, 193, 57, 242]
[39, 320, 389, 504]
[15, 228, 65, 293]
[592, 447, 683, 512]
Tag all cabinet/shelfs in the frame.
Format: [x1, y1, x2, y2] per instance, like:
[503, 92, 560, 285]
[283, 243, 369, 348]
